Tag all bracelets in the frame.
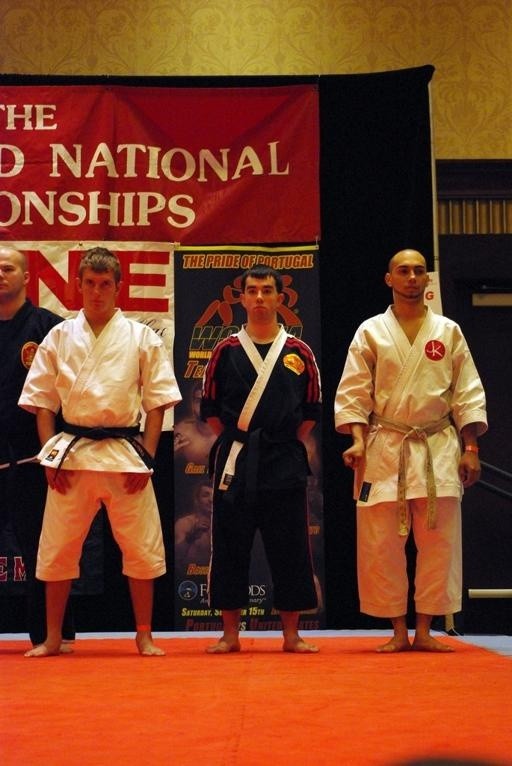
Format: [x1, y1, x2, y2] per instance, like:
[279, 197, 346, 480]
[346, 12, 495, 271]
[464, 444, 480, 453]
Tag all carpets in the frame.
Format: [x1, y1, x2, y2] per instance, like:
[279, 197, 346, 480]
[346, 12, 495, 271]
[0, 635, 512, 766]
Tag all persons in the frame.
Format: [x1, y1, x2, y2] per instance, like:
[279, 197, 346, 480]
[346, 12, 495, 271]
[335, 249, 488, 655]
[0, 245, 75, 655]
[198, 266, 324, 655]
[175, 484, 213, 567]
[174, 385, 218, 472]
[17, 243, 184, 657]
